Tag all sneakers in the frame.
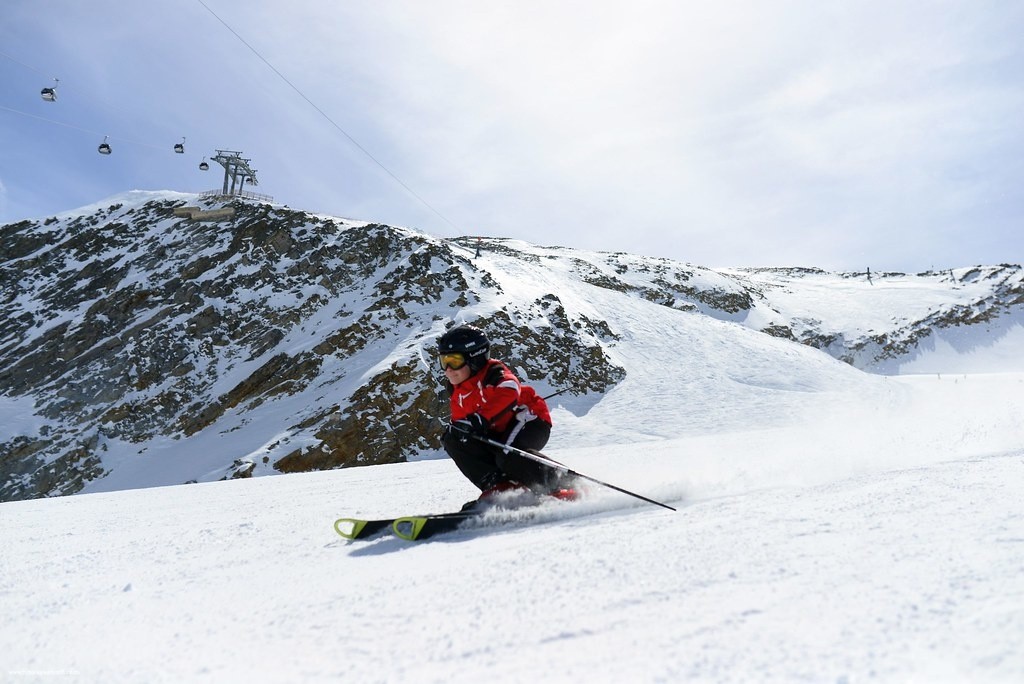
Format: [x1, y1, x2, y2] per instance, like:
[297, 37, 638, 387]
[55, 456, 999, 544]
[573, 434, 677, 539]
[549, 486, 582, 502]
[478, 479, 526, 501]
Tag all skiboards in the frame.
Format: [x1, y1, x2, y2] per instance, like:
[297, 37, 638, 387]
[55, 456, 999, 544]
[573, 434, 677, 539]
[333, 510, 479, 541]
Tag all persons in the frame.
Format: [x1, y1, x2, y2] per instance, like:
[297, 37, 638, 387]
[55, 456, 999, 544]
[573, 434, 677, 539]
[434, 325, 580, 511]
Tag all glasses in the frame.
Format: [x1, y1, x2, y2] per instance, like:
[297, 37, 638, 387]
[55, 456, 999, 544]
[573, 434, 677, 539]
[440, 353, 467, 371]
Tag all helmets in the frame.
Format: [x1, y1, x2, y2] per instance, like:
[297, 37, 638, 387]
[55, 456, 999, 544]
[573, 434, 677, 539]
[438, 324, 491, 378]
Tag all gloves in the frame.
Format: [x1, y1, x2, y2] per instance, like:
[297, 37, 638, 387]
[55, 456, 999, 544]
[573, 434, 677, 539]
[448, 412, 489, 450]
